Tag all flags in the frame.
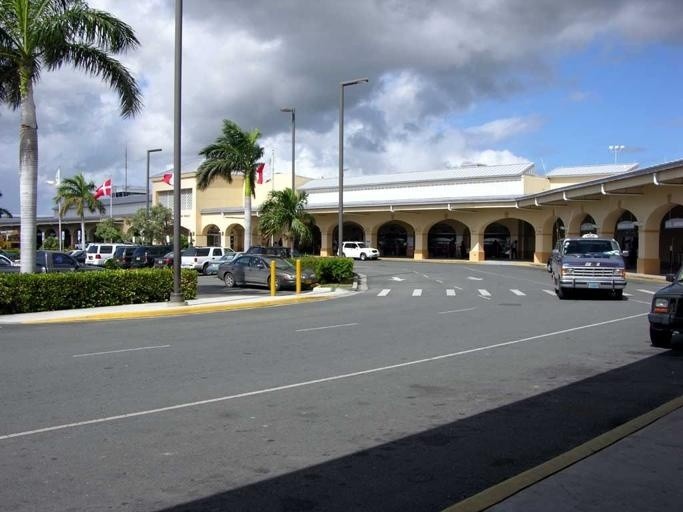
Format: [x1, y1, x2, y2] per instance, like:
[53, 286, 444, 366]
[162, 174, 174, 185]
[256, 157, 272, 185]
[95, 179, 112, 200]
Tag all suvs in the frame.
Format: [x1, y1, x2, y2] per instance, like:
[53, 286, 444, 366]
[338, 240, 380, 262]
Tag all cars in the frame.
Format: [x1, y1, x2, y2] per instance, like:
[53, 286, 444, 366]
[550, 232, 628, 301]
[547, 237, 564, 273]
[648, 262, 682, 352]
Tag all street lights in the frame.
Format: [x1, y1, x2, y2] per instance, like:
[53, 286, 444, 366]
[144, 146, 164, 245]
[338, 77, 374, 259]
[281, 106, 298, 258]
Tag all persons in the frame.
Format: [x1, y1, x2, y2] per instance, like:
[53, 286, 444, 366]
[510, 240, 518, 259]
